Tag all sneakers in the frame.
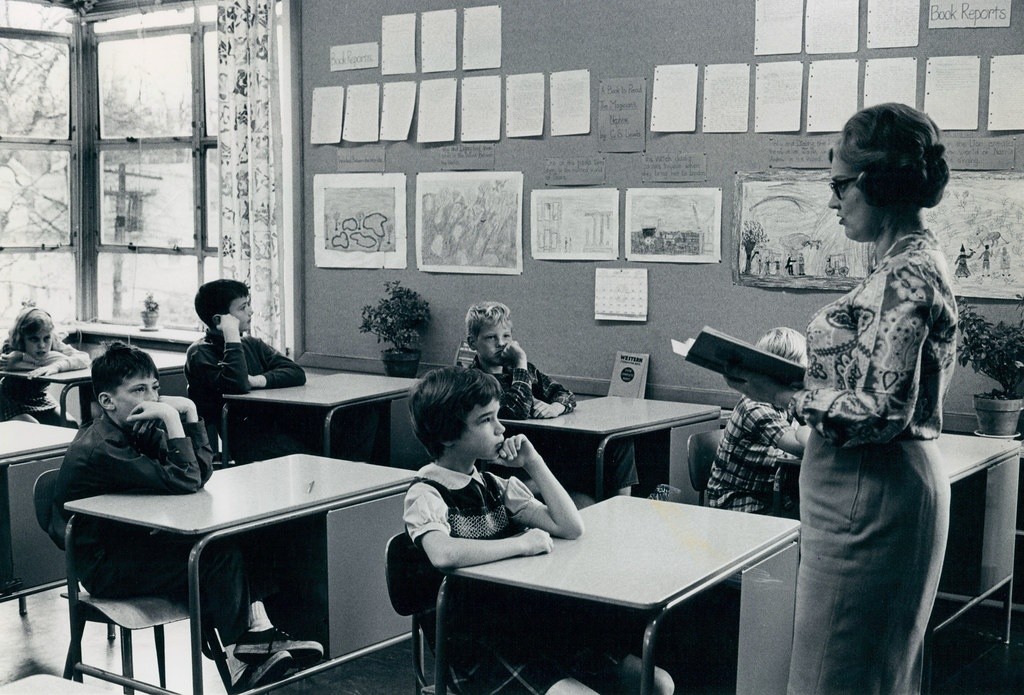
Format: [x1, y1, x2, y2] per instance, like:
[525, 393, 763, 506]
[234, 626, 323, 667]
[234, 650, 293, 695]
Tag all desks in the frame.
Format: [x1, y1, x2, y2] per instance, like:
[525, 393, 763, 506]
[932, 432, 1022, 646]
[0, 418, 118, 643]
[498, 393, 721, 504]
[427, 494, 802, 695]
[219, 371, 439, 466]
[66, 451, 424, 695]
[0, 341, 185, 425]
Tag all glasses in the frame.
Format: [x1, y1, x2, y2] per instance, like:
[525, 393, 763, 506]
[829, 177, 857, 200]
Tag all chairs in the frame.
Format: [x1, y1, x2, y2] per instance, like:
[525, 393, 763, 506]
[687, 425, 725, 507]
[32, 467, 193, 695]
[385, 525, 438, 695]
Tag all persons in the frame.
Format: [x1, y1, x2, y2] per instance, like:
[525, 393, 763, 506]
[707, 327, 806, 518]
[185, 279, 306, 460]
[0, 307, 89, 429]
[466, 301, 638, 496]
[725, 103, 959, 695]
[51, 342, 324, 689]
[402, 366, 675, 695]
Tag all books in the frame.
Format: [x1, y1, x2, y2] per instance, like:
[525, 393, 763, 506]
[671, 325, 806, 387]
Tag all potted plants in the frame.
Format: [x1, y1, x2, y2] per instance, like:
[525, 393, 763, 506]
[360, 277, 429, 375]
[138, 290, 160, 327]
[956, 292, 1024, 437]
